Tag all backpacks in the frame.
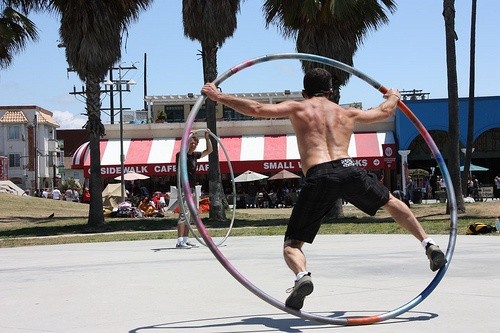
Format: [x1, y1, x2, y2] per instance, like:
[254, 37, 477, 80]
[465, 222, 493, 235]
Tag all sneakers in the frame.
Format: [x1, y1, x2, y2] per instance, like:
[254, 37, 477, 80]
[425, 241, 448, 272]
[176, 242, 197, 249]
[284, 272, 313, 311]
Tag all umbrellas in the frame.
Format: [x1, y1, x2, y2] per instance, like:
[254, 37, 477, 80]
[114, 171, 150, 200]
[101, 183, 126, 206]
[460, 163, 489, 183]
[230, 169, 269, 195]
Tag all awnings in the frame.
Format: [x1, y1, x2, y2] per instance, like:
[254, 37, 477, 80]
[83, 132, 385, 179]
[71, 141, 89, 169]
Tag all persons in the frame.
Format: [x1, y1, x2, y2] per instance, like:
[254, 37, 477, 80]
[269, 170, 301, 195]
[24, 186, 90, 205]
[175, 128, 213, 249]
[380, 175, 500, 200]
[106, 181, 303, 218]
[201, 68, 448, 312]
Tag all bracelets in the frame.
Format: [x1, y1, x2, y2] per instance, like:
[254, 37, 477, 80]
[217, 92, 223, 104]
[388, 94, 401, 101]
